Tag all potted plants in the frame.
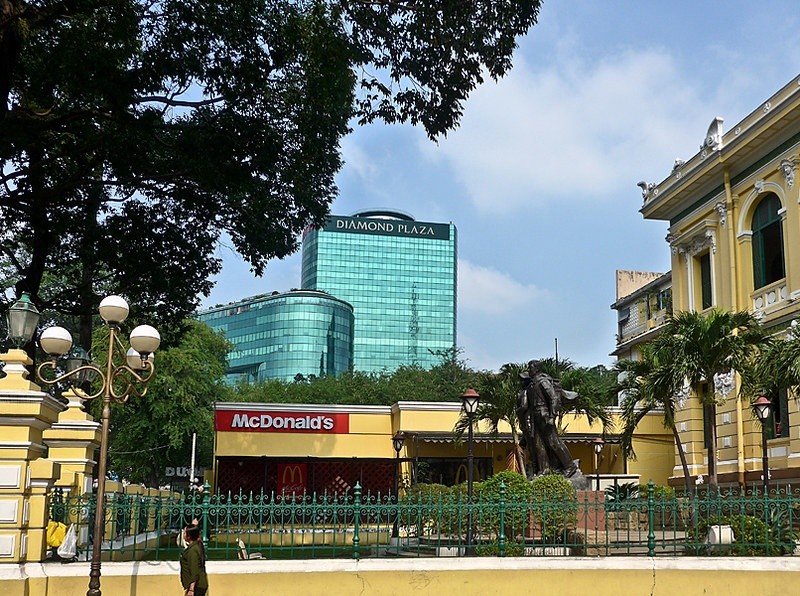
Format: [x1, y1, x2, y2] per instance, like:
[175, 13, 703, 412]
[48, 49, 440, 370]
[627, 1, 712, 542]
[639, 481, 685, 532]
[603, 481, 649, 530]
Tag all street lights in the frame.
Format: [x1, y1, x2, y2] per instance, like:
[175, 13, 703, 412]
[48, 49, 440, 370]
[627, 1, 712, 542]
[390, 430, 409, 538]
[752, 396, 775, 523]
[593, 438, 604, 490]
[460, 387, 485, 556]
[37, 293, 166, 596]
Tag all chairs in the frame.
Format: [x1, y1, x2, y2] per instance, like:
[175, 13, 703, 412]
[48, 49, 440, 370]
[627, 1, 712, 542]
[235, 538, 262, 559]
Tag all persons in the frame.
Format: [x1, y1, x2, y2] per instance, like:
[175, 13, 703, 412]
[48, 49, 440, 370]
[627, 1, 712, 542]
[180, 524, 207, 596]
[515, 360, 577, 478]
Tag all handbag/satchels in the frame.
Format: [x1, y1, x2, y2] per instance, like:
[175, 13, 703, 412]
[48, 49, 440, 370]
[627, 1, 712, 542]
[45, 521, 67, 547]
[57, 524, 78, 558]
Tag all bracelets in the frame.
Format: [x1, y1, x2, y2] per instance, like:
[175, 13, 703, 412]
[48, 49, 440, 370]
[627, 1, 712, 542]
[189, 590, 195, 592]
